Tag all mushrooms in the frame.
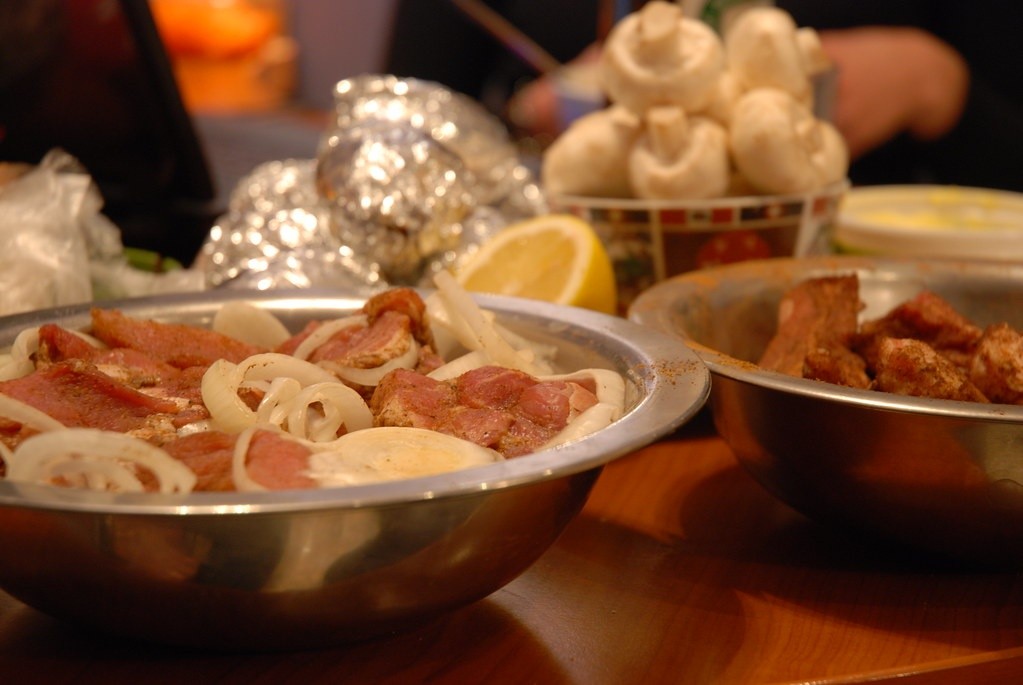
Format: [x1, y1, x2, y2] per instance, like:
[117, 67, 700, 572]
[543, 4, 847, 198]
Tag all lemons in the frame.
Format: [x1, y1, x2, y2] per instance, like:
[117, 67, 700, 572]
[458, 213, 617, 315]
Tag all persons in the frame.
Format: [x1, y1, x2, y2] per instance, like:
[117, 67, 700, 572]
[385, 0, 1023, 201]
[0, 0, 223, 270]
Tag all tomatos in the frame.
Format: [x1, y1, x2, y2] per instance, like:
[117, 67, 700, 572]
[692, 230, 773, 270]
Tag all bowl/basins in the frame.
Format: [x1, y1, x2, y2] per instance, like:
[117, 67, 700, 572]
[629, 255, 1023, 561]
[830, 182, 1022, 265]
[543, 178, 852, 316]
[0, 287, 714, 645]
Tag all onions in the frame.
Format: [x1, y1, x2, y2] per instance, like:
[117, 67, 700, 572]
[0, 302, 623, 493]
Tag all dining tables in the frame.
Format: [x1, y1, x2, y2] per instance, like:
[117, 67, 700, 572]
[0, 439, 1023, 684]
